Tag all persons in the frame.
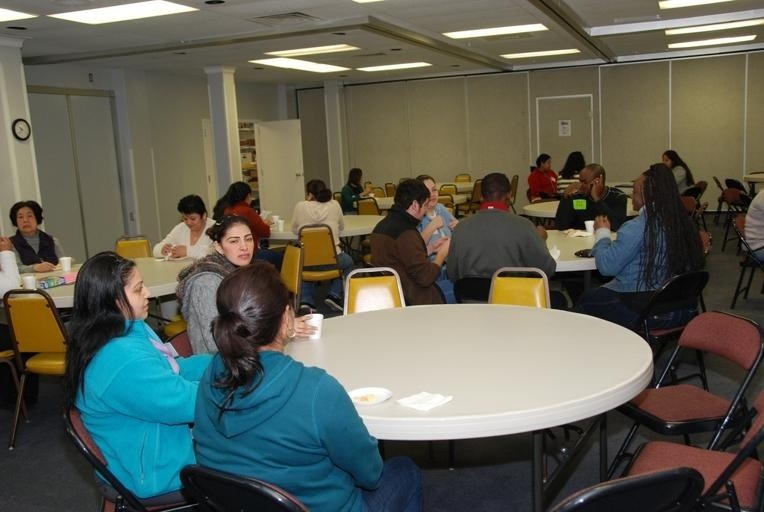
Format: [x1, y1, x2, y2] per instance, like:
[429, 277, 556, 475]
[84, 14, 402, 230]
[0, 235, 20, 299]
[740, 187, 764, 263]
[8, 199, 67, 273]
[66, 149, 706, 512]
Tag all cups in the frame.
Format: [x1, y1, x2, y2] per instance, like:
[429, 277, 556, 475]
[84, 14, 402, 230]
[276, 220, 285, 232]
[59, 256, 72, 271]
[22, 276, 35, 289]
[302, 313, 324, 340]
[584, 220, 595, 233]
[368, 194, 375, 201]
[272, 215, 280, 224]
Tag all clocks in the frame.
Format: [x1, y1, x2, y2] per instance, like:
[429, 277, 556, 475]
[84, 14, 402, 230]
[12, 119, 30, 140]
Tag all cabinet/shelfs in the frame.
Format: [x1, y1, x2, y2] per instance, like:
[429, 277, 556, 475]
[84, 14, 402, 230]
[238, 119, 304, 233]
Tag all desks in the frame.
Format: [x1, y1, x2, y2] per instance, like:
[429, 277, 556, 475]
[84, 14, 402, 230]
[282, 301, 655, 508]
[20, 255, 194, 308]
[522, 198, 640, 229]
[258, 215, 386, 260]
[546, 229, 617, 292]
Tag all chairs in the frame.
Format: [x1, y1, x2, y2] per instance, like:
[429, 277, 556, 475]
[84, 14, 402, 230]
[1, 172, 764, 512]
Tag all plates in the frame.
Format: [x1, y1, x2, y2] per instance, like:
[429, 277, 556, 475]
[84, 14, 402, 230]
[347, 387, 392, 407]
